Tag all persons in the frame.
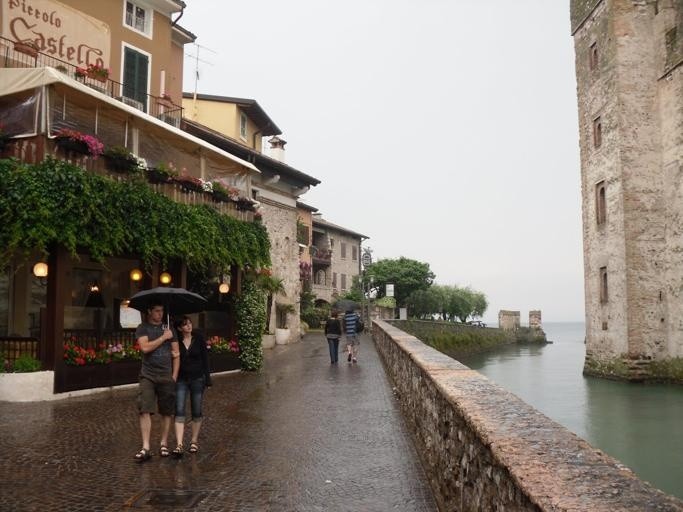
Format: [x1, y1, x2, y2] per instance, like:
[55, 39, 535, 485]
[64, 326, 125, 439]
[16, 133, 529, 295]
[342, 309, 360, 363]
[324, 312, 342, 364]
[170, 315, 212, 454]
[133, 300, 180, 460]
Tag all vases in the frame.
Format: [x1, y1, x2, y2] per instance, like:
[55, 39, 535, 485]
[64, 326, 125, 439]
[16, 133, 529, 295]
[54, 136, 89, 160]
[89, 72, 106, 82]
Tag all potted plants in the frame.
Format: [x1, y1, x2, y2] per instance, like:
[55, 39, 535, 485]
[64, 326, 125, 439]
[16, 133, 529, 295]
[103, 142, 228, 201]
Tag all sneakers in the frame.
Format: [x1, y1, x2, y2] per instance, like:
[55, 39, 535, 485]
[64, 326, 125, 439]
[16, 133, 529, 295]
[330, 352, 358, 364]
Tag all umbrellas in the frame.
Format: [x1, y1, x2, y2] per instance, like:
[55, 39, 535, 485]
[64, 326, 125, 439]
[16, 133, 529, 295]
[126, 286, 209, 331]
[331, 300, 361, 312]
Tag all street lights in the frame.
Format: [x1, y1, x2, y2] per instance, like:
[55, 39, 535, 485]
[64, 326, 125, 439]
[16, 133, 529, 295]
[359, 278, 364, 322]
[368, 275, 374, 335]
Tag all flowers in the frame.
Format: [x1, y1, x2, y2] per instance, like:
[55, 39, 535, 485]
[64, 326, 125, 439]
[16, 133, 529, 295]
[91, 64, 110, 76]
[54, 127, 104, 160]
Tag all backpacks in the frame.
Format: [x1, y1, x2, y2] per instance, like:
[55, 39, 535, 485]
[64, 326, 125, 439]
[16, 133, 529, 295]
[353, 315, 364, 333]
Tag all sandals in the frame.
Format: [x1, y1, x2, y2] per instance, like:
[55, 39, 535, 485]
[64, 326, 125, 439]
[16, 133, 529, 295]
[171, 445, 184, 455]
[158, 445, 169, 457]
[188, 441, 200, 452]
[133, 447, 152, 459]
